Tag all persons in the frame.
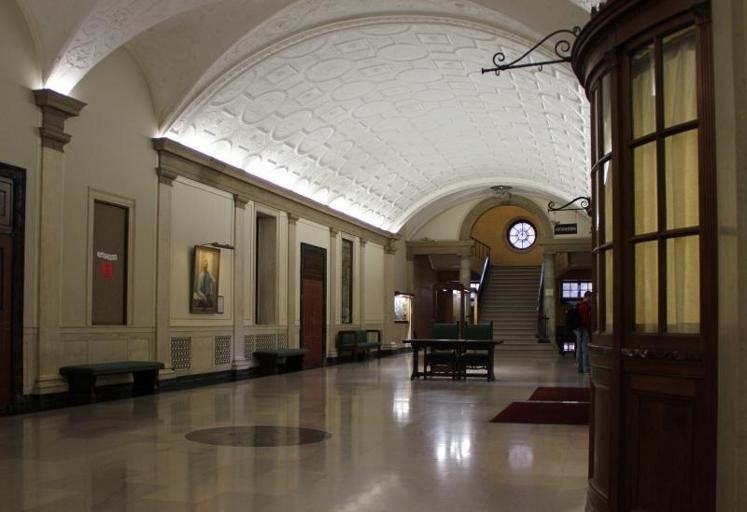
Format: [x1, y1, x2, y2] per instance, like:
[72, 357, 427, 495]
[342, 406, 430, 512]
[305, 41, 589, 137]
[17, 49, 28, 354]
[573, 290, 594, 375]
[563, 302, 577, 356]
[196, 257, 216, 307]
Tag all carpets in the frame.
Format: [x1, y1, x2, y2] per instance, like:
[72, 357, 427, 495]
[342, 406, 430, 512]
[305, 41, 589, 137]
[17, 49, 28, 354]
[487, 385, 590, 424]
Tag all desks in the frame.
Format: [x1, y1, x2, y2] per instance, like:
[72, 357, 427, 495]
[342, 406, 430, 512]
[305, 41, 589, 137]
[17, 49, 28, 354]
[400, 337, 503, 381]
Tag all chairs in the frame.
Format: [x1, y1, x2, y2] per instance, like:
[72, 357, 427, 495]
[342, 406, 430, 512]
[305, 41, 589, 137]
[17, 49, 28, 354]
[425, 320, 494, 381]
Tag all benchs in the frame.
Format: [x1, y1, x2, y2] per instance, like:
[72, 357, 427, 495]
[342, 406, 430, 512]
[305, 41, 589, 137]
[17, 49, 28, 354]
[58, 362, 163, 401]
[334, 329, 380, 360]
[253, 349, 310, 373]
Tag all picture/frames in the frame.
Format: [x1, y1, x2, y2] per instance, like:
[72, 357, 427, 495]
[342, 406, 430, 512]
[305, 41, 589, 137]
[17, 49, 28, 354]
[190, 245, 219, 314]
